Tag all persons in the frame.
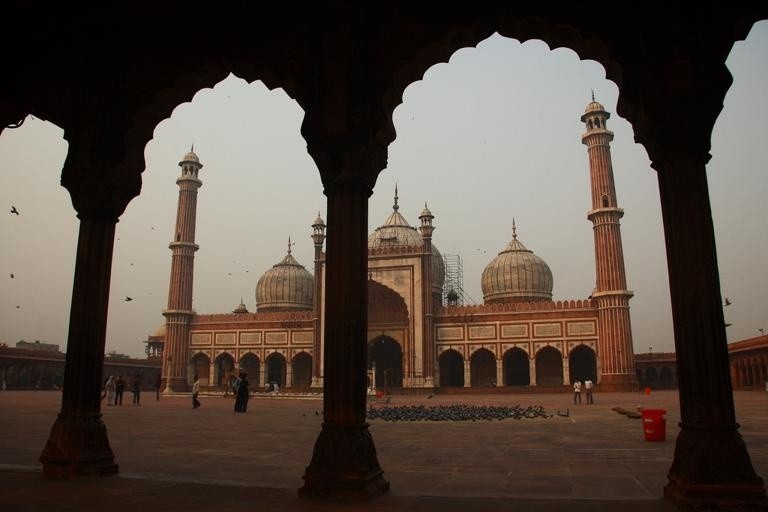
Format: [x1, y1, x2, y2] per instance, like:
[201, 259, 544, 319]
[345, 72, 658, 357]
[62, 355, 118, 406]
[191, 375, 201, 409]
[105, 375, 116, 405]
[101, 375, 110, 399]
[366, 386, 372, 396]
[573, 377, 582, 404]
[585, 376, 594, 404]
[263, 377, 282, 393]
[154, 373, 162, 401]
[371, 386, 377, 396]
[221, 371, 250, 414]
[113, 375, 125, 405]
[131, 374, 142, 406]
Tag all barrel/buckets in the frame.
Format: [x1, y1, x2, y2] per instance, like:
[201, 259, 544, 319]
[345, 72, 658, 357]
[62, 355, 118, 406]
[641, 409, 666, 440]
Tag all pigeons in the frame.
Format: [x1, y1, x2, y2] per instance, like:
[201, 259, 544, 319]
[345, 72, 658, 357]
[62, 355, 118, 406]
[364, 402, 571, 424]
[723, 297, 732, 306]
[125, 297, 133, 301]
[11, 205, 19, 215]
